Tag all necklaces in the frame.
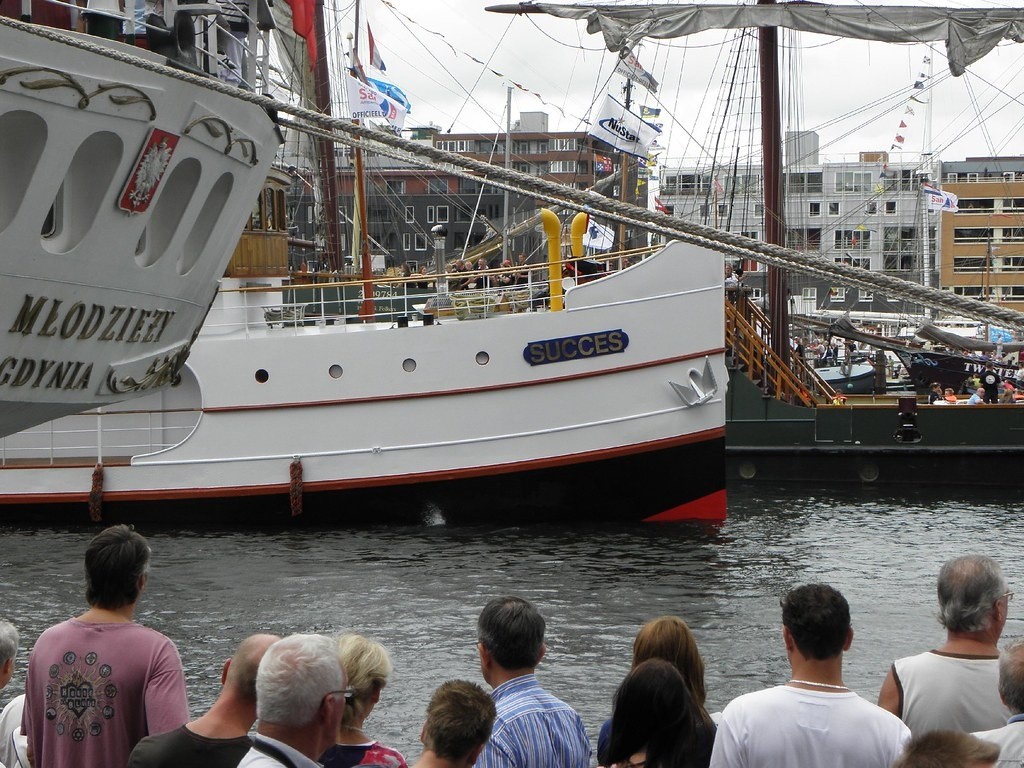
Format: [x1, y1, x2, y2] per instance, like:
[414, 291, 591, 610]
[789, 679, 851, 692]
[342, 724, 365, 733]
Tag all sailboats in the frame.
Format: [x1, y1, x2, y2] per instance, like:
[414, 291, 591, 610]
[245, 1, 1024, 486]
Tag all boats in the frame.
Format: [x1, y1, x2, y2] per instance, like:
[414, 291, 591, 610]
[1, 2, 733, 540]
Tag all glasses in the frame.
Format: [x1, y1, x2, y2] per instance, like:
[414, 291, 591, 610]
[993, 590, 1014, 607]
[321, 686, 354, 709]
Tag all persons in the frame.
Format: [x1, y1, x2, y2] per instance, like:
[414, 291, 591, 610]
[970, 640, 1024, 768]
[596, 615, 719, 768]
[708, 584, 910, 768]
[236, 634, 354, 768]
[891, 729, 1001, 768]
[20, 524, 190, 768]
[725, 262, 1024, 406]
[878, 554, 1014, 738]
[398, 253, 531, 291]
[317, 635, 409, 768]
[411, 679, 497, 768]
[470, 594, 593, 768]
[126, 634, 282, 768]
[0, 621, 34, 768]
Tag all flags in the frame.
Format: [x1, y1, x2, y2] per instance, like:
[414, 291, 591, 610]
[923, 183, 959, 212]
[581, 218, 615, 249]
[344, 21, 411, 136]
[588, 46, 662, 172]
